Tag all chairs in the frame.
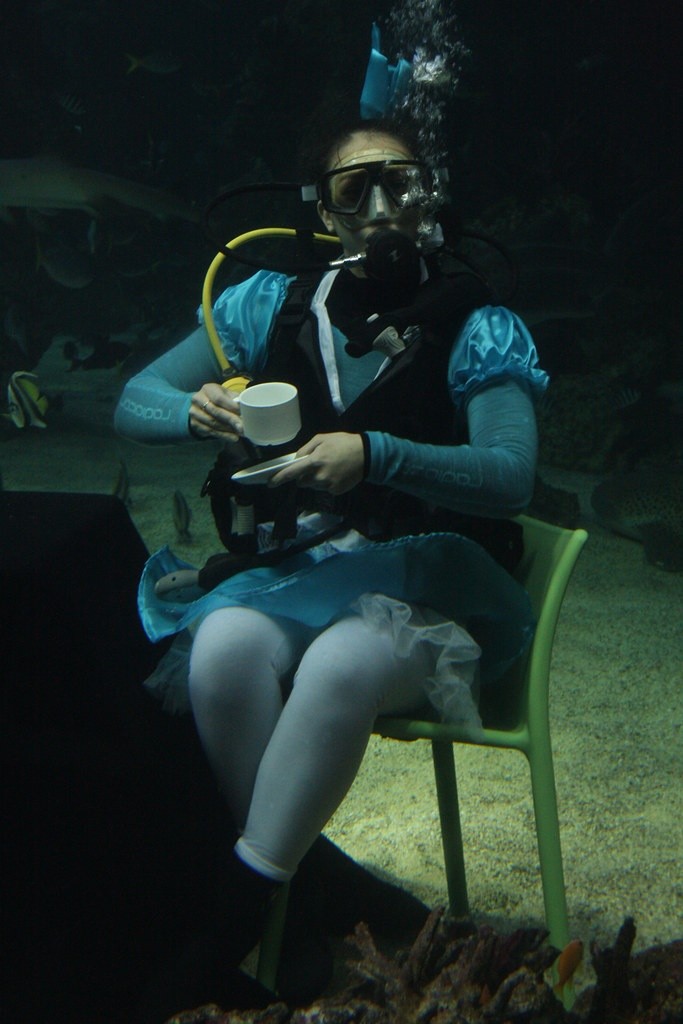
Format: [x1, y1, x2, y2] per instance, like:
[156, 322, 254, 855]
[258, 513, 591, 953]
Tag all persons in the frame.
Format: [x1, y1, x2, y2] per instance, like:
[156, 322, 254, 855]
[117, 124, 549, 1007]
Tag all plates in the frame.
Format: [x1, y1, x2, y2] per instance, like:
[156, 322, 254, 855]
[232, 452, 309, 485]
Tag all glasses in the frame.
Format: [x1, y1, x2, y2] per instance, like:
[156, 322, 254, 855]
[316, 159, 432, 217]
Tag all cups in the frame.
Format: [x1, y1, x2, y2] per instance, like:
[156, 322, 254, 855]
[233, 382, 301, 446]
